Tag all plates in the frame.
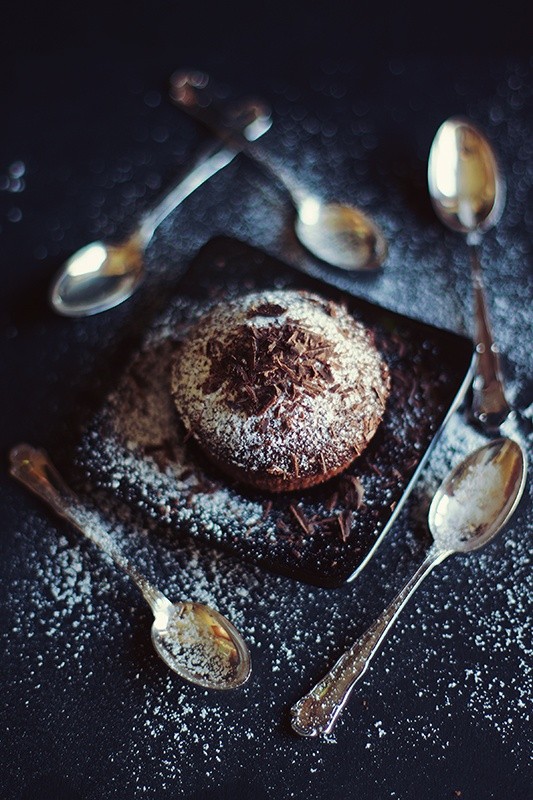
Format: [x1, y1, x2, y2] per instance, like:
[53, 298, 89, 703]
[72, 226, 480, 585]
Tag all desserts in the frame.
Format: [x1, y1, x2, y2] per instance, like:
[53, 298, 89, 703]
[175, 282, 397, 495]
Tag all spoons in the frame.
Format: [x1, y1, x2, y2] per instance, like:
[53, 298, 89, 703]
[51, 102, 272, 315]
[289, 436, 526, 736]
[169, 70, 394, 275]
[8, 443, 253, 689]
[428, 117, 515, 434]
